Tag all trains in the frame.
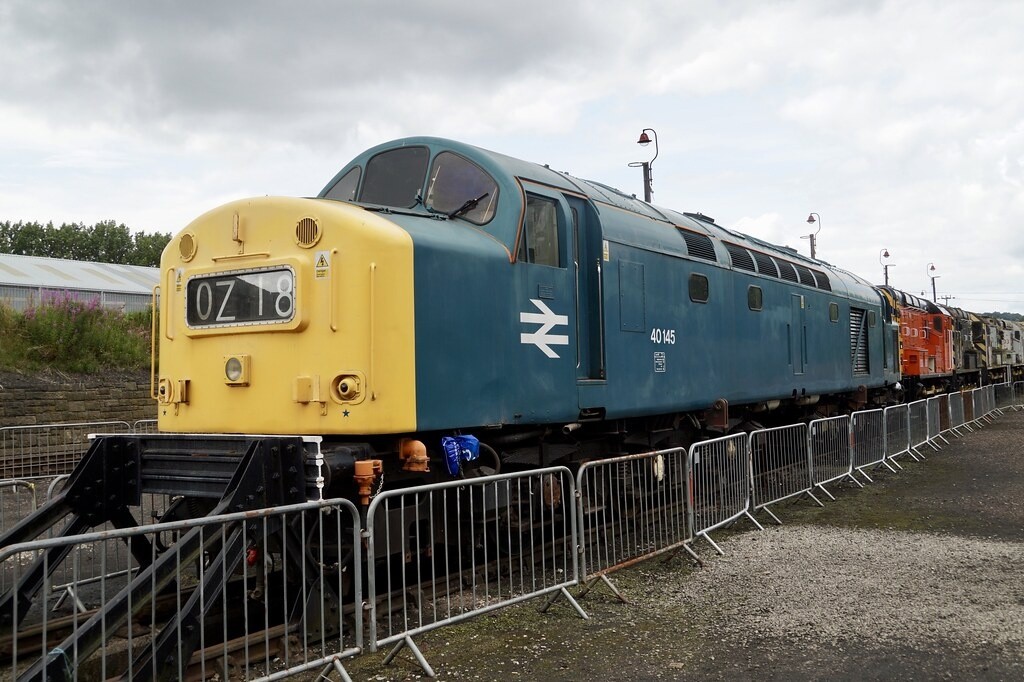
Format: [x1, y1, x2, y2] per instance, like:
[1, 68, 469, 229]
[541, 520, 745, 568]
[1, 135, 1024, 682]
